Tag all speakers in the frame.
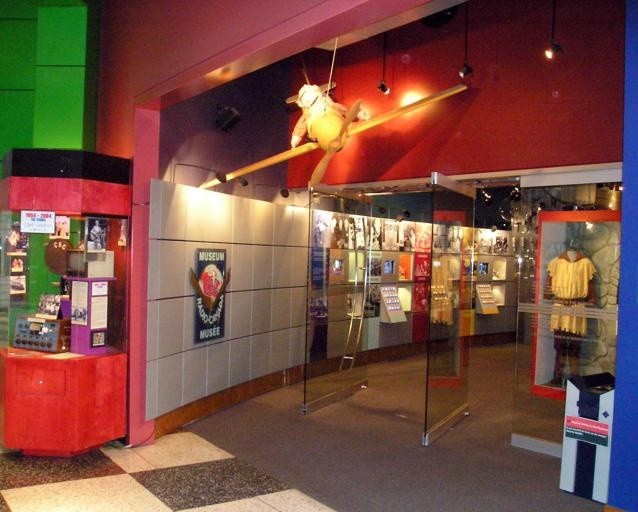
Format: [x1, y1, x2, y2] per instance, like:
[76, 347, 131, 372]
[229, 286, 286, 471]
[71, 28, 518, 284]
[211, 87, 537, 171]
[213, 106, 243, 131]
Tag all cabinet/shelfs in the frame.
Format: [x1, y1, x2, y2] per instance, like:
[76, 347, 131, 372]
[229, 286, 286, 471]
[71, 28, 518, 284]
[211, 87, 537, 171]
[0, 149, 133, 457]
[307, 210, 432, 363]
[430, 223, 516, 340]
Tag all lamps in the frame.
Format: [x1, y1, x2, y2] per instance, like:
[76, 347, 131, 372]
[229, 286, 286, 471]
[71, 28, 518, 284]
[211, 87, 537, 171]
[376, 33, 390, 95]
[173, 163, 228, 184]
[458, 0, 473, 79]
[545, 0, 563, 60]
[216, 104, 241, 132]
[479, 188, 492, 202]
[256, 184, 289, 197]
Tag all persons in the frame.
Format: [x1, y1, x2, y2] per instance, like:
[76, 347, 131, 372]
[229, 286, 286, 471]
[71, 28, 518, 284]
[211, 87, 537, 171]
[544, 248, 597, 385]
[91, 221, 101, 250]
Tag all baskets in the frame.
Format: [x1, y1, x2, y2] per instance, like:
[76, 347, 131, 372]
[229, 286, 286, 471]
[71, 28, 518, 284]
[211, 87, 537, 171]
[12, 315, 71, 353]
